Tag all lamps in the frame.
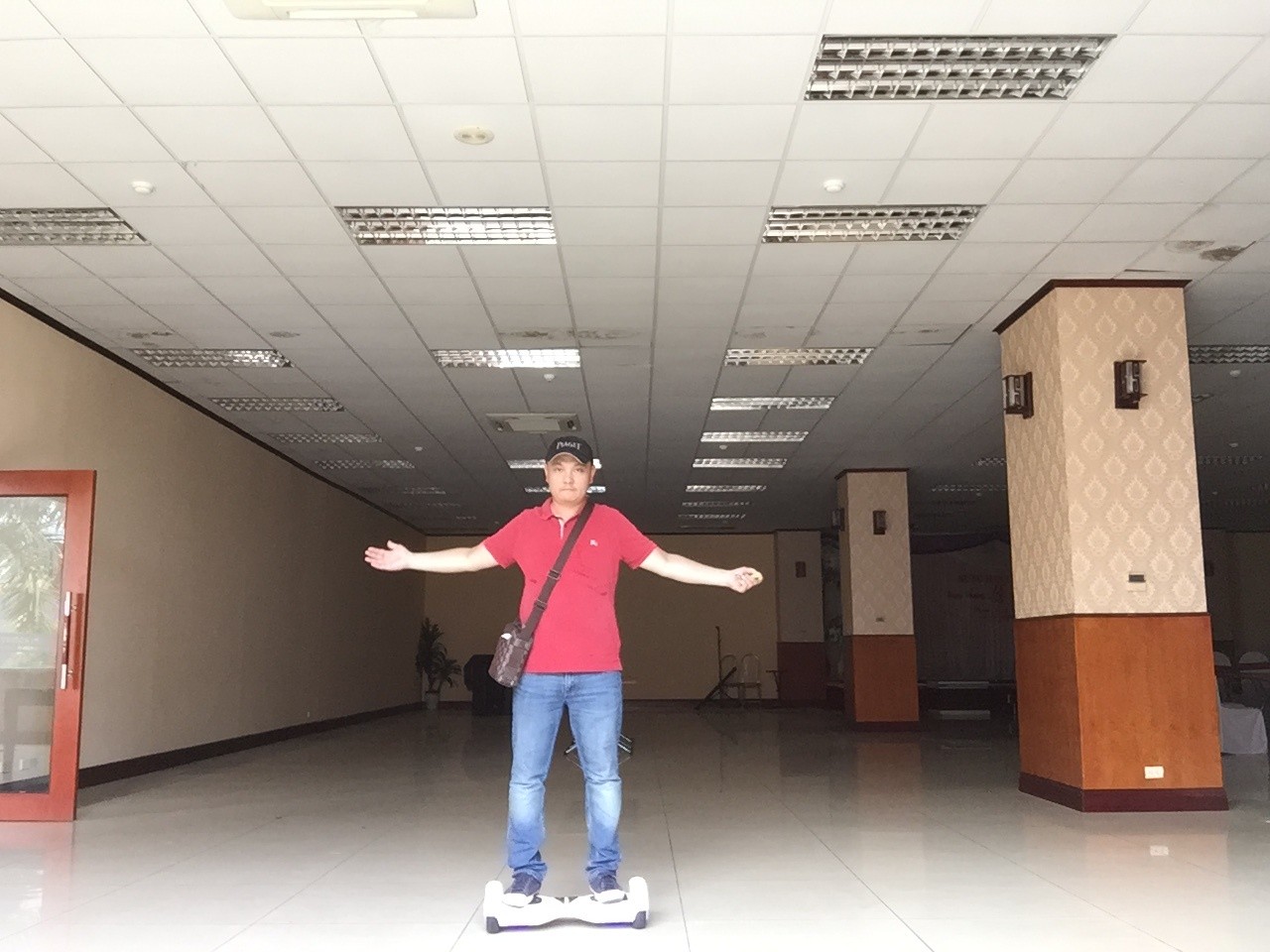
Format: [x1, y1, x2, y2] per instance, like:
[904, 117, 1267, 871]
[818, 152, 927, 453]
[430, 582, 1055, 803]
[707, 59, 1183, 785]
[1002, 372, 1034, 418]
[795, 561, 806, 577]
[1114, 360, 1147, 409]
[873, 510, 887, 535]
[831, 508, 845, 532]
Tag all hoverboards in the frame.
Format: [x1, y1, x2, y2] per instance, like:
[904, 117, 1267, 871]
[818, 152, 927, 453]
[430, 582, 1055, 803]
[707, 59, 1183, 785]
[485, 876, 649, 934]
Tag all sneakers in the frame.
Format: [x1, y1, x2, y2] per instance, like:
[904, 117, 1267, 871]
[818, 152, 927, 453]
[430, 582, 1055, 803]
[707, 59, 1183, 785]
[590, 874, 624, 903]
[503, 875, 541, 907]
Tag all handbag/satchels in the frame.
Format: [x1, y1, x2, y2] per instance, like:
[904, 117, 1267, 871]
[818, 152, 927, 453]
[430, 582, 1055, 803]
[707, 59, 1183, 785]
[489, 622, 533, 687]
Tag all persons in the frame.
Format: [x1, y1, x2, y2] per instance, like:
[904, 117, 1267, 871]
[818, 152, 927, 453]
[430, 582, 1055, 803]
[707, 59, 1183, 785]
[365, 436, 763, 907]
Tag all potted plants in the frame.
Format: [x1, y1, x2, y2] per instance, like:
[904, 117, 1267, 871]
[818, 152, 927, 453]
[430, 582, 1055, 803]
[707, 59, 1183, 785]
[413, 616, 461, 709]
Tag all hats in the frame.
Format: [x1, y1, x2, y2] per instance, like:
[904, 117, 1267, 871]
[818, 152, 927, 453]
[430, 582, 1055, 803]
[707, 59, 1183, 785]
[546, 436, 593, 465]
